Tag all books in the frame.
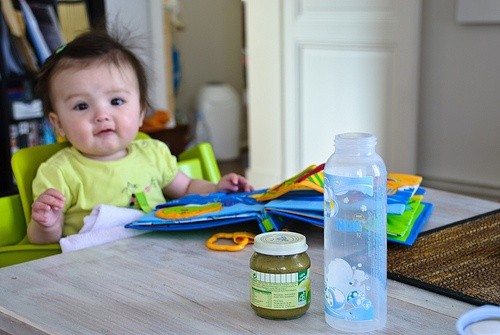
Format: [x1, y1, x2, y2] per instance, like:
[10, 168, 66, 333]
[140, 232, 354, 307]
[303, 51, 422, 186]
[123, 161, 434, 246]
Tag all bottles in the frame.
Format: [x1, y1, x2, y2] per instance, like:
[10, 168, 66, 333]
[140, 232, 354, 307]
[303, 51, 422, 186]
[324, 133, 389, 332]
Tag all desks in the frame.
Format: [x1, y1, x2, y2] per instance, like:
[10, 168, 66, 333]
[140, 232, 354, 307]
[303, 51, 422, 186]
[0, 188, 500, 335]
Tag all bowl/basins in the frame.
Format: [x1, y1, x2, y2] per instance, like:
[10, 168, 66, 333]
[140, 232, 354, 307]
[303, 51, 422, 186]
[456, 306, 500, 335]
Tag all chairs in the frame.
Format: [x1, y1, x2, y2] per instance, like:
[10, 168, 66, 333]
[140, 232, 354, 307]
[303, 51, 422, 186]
[11, 132, 153, 245]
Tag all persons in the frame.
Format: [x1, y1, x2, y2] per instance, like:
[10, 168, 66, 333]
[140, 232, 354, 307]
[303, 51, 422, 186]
[26, 31, 254, 244]
